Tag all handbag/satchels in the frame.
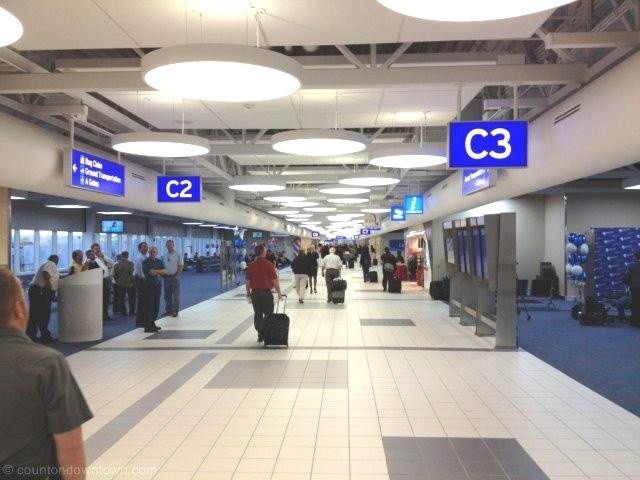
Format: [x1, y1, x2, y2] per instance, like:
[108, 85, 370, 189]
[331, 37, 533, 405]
[332, 278, 347, 290]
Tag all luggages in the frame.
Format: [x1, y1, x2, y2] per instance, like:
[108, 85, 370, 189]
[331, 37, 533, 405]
[390, 278, 402, 293]
[370, 271, 378, 282]
[263, 313, 290, 347]
[331, 275, 344, 303]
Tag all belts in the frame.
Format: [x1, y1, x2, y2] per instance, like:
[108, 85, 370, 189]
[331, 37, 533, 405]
[252, 288, 271, 291]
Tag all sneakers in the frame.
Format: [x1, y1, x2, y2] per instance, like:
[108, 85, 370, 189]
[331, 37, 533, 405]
[310, 292, 312, 294]
[299, 300, 303, 303]
[388, 290, 391, 292]
[314, 290, 317, 293]
[258, 338, 262, 342]
[327, 300, 331, 303]
[383, 289, 387, 291]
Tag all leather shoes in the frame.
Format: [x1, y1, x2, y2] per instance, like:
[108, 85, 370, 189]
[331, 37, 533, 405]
[103, 317, 115, 321]
[162, 312, 178, 317]
[145, 327, 161, 332]
[41, 336, 58, 343]
[129, 312, 137, 316]
[121, 313, 128, 316]
[31, 337, 40, 341]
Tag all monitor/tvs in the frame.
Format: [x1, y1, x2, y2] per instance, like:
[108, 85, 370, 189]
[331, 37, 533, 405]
[100, 220, 125, 234]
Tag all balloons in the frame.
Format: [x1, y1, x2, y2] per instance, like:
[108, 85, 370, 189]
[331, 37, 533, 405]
[565, 232, 589, 286]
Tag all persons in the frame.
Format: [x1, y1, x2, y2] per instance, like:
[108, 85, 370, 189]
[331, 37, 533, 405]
[132, 240, 150, 328]
[244, 244, 282, 343]
[80, 248, 105, 274]
[183, 252, 189, 260]
[142, 245, 168, 333]
[213, 253, 218, 257]
[161, 240, 184, 317]
[90, 243, 116, 321]
[395, 250, 404, 264]
[304, 245, 320, 294]
[290, 249, 309, 303]
[315, 240, 379, 283]
[68, 249, 87, 275]
[205, 251, 212, 258]
[110, 253, 127, 316]
[24, 254, 69, 343]
[0, 265, 93, 480]
[193, 251, 199, 259]
[113, 250, 139, 316]
[321, 246, 343, 303]
[620, 248, 640, 327]
[380, 246, 397, 292]
